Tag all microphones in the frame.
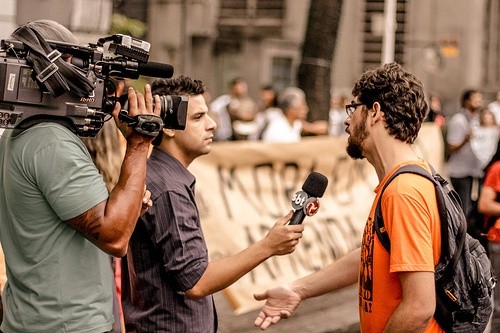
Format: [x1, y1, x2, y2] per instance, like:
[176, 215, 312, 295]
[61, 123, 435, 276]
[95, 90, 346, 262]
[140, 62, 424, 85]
[288, 172, 328, 225]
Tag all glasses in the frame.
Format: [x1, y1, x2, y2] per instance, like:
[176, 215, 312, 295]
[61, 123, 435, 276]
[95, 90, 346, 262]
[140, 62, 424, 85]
[345, 101, 370, 116]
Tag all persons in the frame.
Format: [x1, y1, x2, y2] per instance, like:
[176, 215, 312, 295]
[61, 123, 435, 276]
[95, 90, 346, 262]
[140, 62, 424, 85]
[208, 77, 447, 143]
[254, 62, 497, 333]
[0, 19, 162, 333]
[121, 75, 305, 332]
[445, 91, 500, 333]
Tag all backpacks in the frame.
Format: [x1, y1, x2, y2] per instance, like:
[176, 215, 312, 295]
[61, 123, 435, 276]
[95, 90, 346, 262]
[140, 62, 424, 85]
[430, 171, 498, 333]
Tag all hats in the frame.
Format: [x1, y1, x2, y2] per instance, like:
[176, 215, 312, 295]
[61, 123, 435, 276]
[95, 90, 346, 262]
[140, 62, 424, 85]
[29, 19, 80, 46]
